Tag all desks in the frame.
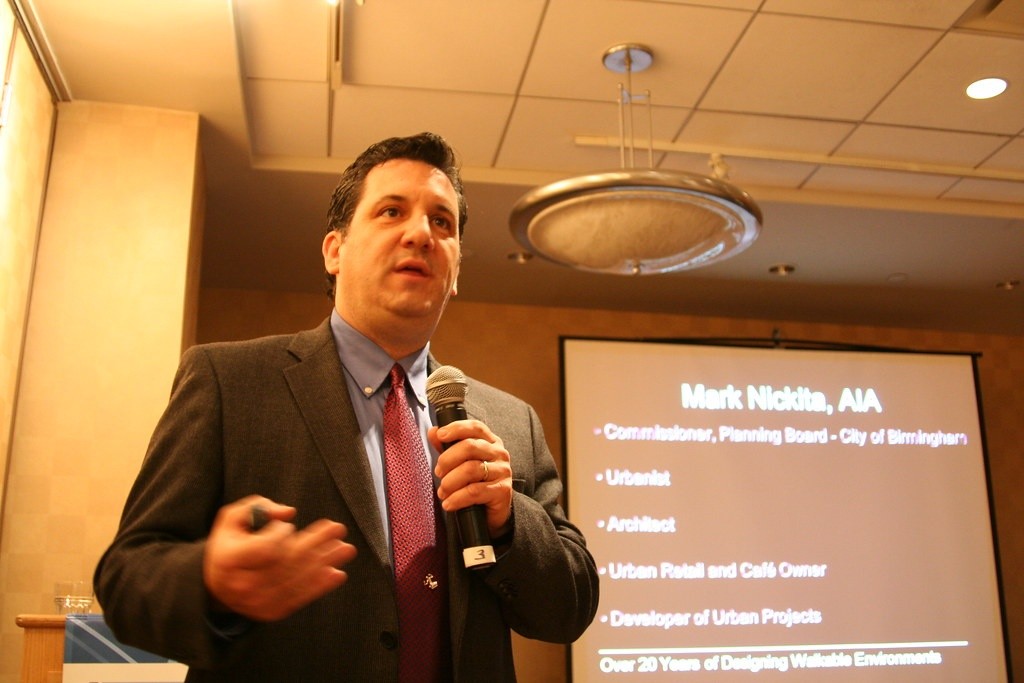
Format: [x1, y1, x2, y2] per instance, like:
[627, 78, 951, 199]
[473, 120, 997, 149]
[16, 614, 74, 683]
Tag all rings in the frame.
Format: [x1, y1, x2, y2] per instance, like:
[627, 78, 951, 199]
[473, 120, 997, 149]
[484, 460, 489, 480]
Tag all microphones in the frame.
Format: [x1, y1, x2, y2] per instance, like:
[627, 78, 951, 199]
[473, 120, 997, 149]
[426, 365, 497, 572]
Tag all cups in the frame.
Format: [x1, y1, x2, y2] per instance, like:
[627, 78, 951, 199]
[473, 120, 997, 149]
[52, 579, 95, 616]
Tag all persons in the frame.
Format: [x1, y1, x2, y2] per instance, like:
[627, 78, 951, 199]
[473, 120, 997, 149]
[92, 132, 600, 683]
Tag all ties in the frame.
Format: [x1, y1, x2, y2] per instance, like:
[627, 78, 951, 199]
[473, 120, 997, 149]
[384, 363, 447, 682]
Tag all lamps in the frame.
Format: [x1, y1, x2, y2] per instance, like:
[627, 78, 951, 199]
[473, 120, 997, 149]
[508, 41, 765, 277]
